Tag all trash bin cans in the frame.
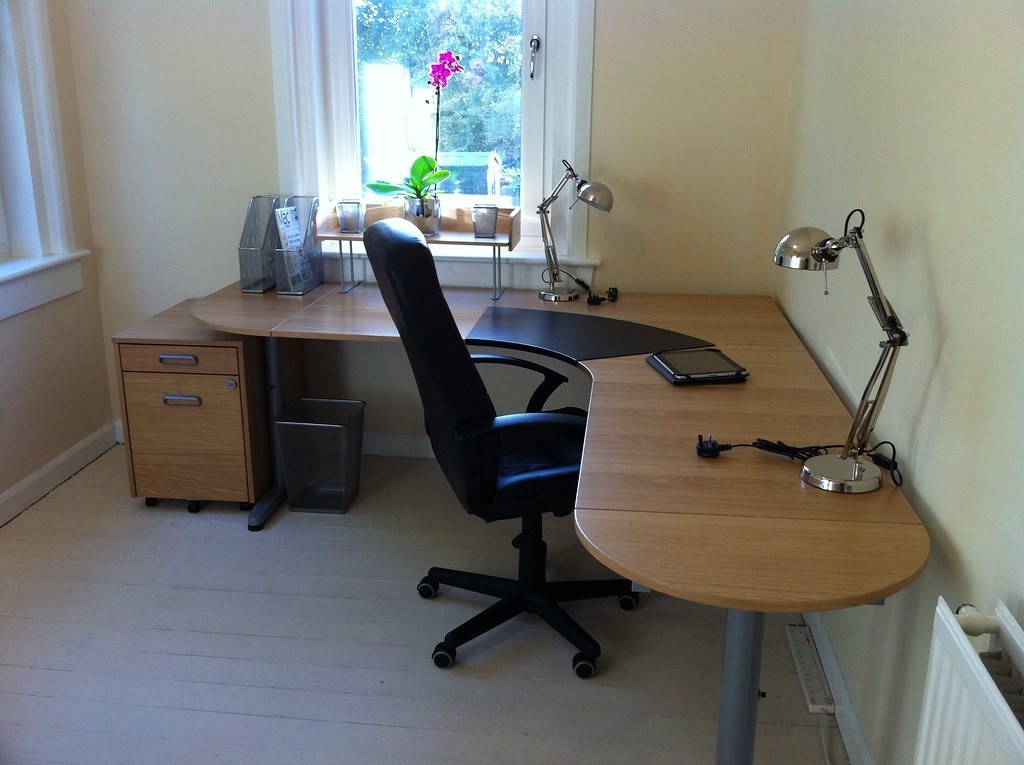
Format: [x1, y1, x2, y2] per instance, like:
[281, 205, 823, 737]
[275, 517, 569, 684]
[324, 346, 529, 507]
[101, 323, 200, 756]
[274, 396, 368, 514]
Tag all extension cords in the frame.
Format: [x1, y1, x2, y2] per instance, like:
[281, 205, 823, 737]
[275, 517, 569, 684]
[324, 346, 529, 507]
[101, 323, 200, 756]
[788, 623, 835, 714]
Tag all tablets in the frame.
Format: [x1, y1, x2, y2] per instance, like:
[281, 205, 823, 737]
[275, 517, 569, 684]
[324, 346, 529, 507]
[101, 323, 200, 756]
[646, 348, 750, 386]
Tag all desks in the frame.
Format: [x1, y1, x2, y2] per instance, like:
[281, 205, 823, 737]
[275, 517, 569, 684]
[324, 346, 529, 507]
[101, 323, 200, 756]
[190, 275, 930, 765]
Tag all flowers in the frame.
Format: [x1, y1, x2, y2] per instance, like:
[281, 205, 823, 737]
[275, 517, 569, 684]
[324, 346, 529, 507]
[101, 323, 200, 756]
[364, 50, 465, 216]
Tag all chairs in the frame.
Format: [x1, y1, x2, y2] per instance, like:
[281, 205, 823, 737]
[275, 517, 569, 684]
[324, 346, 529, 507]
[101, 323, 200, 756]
[362, 217, 639, 681]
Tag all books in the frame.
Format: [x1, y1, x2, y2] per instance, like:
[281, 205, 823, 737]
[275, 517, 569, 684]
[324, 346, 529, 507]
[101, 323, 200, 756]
[274, 206, 318, 293]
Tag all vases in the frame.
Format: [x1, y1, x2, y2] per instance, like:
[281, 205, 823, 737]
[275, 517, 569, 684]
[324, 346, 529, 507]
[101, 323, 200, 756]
[470, 202, 499, 239]
[403, 198, 441, 237]
[335, 198, 366, 234]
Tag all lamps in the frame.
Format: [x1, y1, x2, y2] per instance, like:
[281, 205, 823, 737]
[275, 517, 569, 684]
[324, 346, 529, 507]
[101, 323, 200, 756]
[535, 159, 619, 304]
[696, 209, 912, 495]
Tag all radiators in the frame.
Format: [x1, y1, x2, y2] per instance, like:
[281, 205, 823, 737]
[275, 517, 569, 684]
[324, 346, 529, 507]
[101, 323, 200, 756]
[913, 601, 1024, 765]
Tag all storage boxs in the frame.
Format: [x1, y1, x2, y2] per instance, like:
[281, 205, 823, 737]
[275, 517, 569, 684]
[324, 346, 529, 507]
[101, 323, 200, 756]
[273, 196, 324, 295]
[236, 193, 280, 292]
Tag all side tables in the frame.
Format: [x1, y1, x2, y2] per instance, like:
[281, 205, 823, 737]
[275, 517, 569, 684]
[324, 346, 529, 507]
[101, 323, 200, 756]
[110, 298, 276, 513]
